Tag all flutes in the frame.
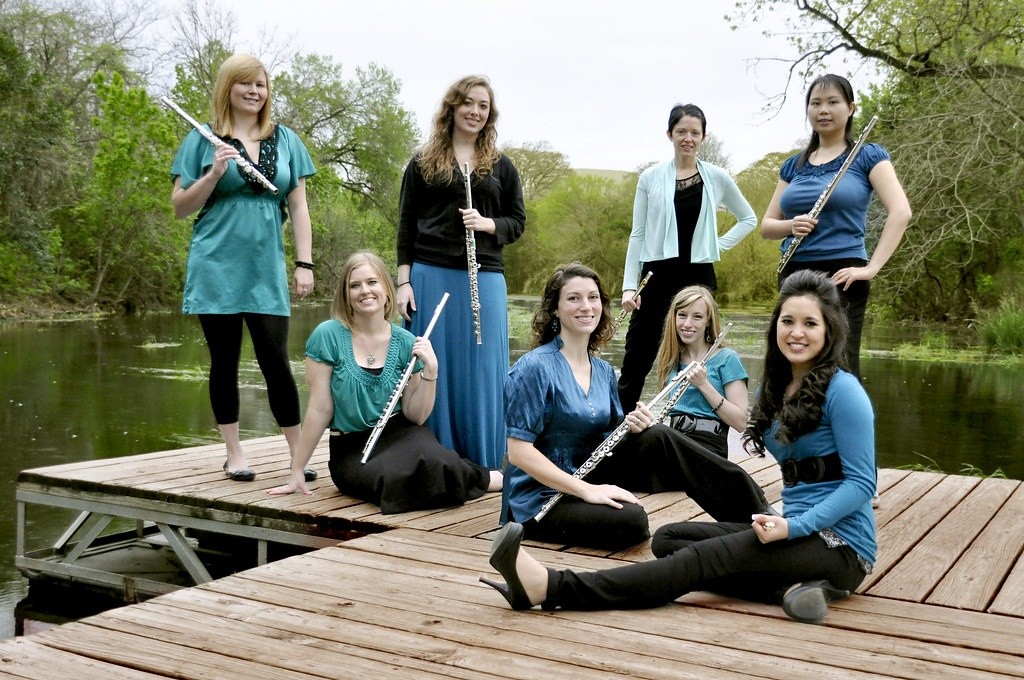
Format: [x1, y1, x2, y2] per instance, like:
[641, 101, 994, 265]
[359, 292, 450, 465]
[158, 95, 279, 195]
[463, 162, 483, 345]
[761, 520, 776, 531]
[535, 361, 698, 523]
[776, 115, 879, 275]
[615, 271, 654, 329]
[658, 321, 735, 425]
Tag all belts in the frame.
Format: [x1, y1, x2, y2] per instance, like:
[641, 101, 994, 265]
[670, 414, 728, 436]
[779, 454, 844, 487]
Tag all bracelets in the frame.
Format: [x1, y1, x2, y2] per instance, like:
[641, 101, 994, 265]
[419, 370, 438, 381]
[712, 397, 724, 412]
[295, 260, 316, 269]
[397, 282, 410, 287]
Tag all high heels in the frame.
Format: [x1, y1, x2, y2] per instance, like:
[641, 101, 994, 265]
[783, 579, 851, 623]
[477, 522, 534, 611]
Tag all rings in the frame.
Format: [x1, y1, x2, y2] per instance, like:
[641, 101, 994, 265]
[220, 152, 224, 156]
[637, 418, 640, 425]
[608, 498, 611, 504]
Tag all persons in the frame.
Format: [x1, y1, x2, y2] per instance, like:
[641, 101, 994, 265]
[617, 104, 758, 416]
[478, 268, 877, 619]
[498, 263, 782, 550]
[395, 74, 527, 470]
[760, 73, 913, 386]
[170, 54, 318, 482]
[265, 250, 504, 514]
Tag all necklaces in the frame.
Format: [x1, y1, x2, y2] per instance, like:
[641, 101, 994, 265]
[361, 337, 376, 365]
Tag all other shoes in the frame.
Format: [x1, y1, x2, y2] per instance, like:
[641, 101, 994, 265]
[223, 460, 256, 482]
[290, 461, 316, 480]
